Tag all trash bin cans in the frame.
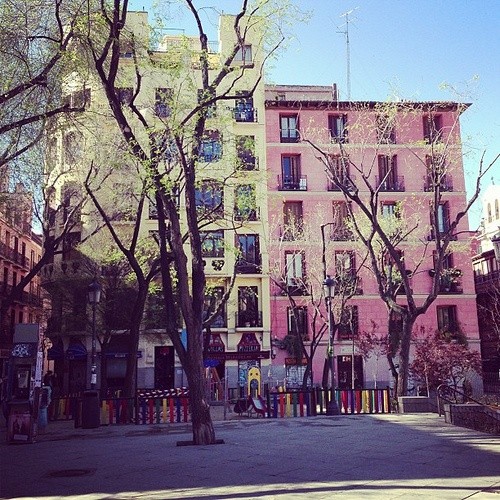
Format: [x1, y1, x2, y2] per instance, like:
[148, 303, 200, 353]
[81, 389, 101, 428]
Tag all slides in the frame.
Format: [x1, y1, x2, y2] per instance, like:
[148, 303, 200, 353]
[250, 395, 267, 413]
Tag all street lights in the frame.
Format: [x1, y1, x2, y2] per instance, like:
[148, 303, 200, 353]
[322, 279, 339, 414]
[90, 277, 101, 391]
